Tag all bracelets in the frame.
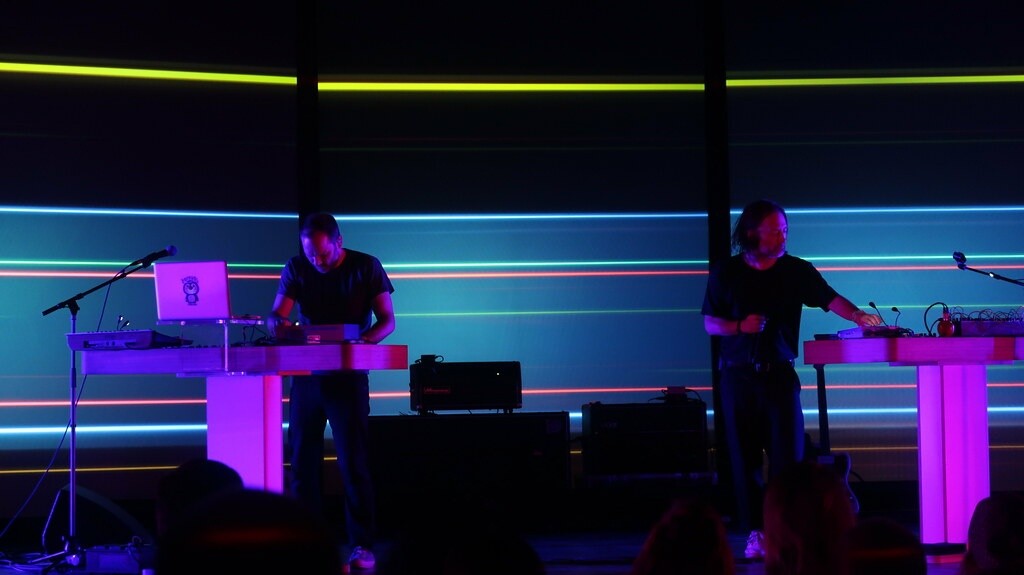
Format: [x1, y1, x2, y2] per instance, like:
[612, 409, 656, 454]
[737, 319, 743, 333]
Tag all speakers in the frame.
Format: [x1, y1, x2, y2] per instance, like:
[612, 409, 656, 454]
[581, 400, 708, 478]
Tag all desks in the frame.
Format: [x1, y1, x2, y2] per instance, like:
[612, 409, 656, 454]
[79, 343, 409, 496]
[803, 335, 1024, 575]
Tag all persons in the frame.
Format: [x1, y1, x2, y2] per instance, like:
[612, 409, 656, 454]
[154, 450, 1024, 575]
[271, 211, 395, 570]
[697, 200, 882, 559]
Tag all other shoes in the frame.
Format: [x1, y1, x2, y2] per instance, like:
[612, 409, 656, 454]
[349, 545, 375, 570]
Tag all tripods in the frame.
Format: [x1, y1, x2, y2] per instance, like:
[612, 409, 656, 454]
[25, 262, 151, 564]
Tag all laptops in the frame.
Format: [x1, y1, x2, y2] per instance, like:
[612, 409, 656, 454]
[154, 260, 263, 320]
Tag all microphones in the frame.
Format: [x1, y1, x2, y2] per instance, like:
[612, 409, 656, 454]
[131, 245, 177, 266]
[749, 310, 767, 362]
[868, 302, 888, 327]
[892, 307, 901, 327]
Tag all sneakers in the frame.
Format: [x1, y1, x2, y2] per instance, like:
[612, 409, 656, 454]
[745, 532, 767, 559]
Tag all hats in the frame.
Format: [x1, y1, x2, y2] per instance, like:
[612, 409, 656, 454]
[155, 489, 342, 575]
[629, 501, 736, 575]
[779, 461, 827, 503]
[969, 492, 1023, 570]
